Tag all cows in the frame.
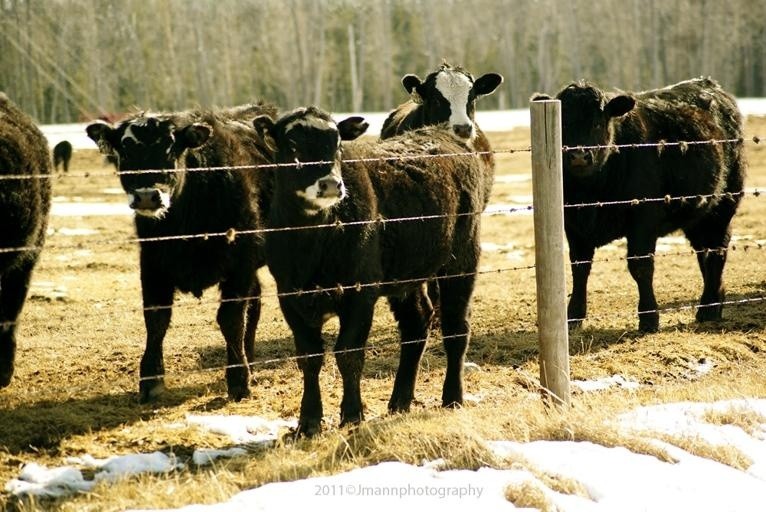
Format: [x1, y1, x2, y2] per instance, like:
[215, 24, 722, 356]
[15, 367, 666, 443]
[0, 90, 53, 388]
[253, 104, 488, 437]
[379, 58, 504, 331]
[529, 76, 747, 333]
[85, 98, 279, 402]
